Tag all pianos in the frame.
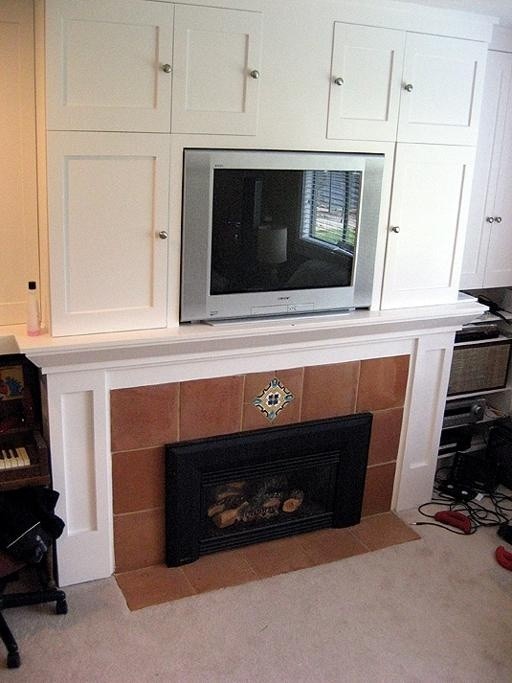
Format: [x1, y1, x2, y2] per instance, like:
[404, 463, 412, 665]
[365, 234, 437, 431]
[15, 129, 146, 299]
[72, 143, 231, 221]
[0, 352, 57, 593]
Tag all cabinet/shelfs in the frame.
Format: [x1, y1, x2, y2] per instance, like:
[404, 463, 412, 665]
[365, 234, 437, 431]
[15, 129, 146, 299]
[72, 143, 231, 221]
[459, 49, 512, 291]
[170, 3, 263, 136]
[397, 32, 488, 147]
[380, 144, 476, 310]
[0, 2, 42, 327]
[325, 21, 406, 142]
[44, 1, 174, 134]
[45, 131, 170, 338]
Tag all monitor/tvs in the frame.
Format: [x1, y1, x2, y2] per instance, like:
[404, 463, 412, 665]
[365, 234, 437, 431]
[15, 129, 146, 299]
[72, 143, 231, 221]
[181, 149, 385, 322]
[446, 339, 512, 401]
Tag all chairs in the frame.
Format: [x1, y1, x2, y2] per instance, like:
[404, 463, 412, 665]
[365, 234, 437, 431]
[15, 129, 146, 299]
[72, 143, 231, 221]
[0, 486, 68, 668]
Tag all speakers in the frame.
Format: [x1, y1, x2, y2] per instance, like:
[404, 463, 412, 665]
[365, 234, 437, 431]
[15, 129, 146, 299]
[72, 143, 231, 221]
[438, 451, 477, 502]
